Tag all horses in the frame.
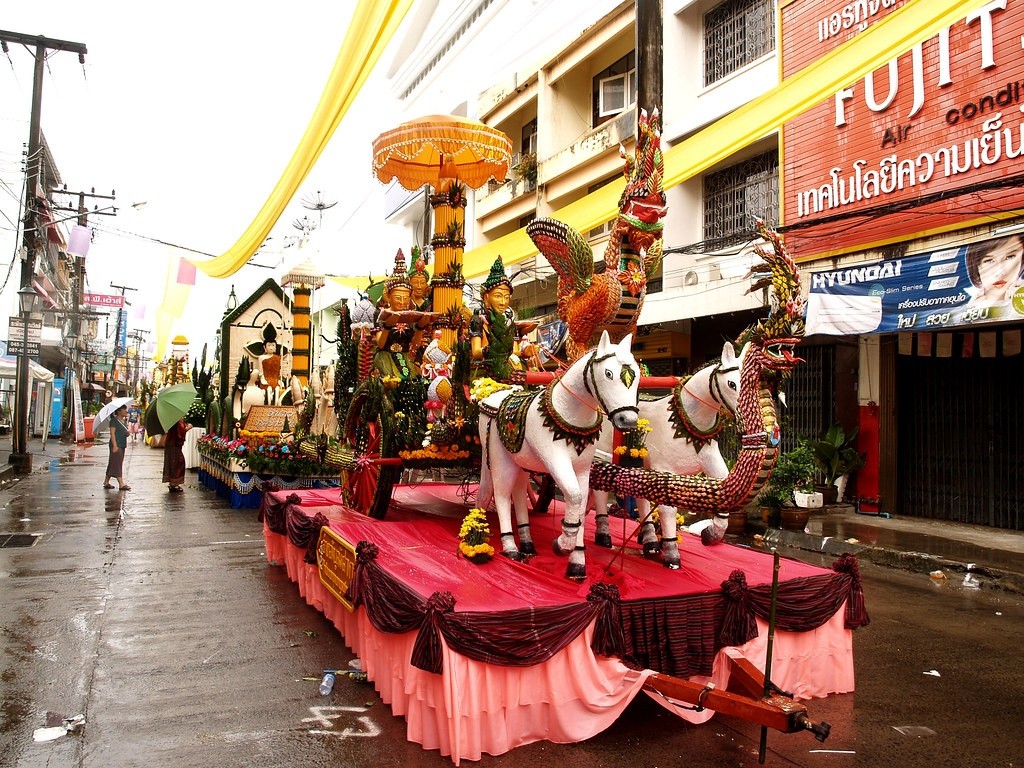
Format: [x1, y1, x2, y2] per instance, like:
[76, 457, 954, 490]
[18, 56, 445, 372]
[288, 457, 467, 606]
[591, 340, 753, 571]
[475, 329, 642, 581]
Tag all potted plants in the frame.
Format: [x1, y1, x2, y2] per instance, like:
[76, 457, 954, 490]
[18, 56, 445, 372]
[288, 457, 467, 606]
[757, 424, 866, 531]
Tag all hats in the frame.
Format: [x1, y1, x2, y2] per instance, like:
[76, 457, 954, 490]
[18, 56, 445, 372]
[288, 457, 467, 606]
[131, 403, 138, 406]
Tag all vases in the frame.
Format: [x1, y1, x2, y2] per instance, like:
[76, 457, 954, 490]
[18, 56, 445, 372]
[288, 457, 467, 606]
[728, 512, 749, 533]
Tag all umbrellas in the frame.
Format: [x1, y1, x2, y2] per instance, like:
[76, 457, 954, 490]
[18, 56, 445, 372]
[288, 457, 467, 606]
[92, 398, 134, 436]
[144, 382, 193, 438]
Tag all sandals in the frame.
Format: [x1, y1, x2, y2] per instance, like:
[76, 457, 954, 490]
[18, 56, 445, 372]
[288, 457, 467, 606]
[169, 484, 183, 492]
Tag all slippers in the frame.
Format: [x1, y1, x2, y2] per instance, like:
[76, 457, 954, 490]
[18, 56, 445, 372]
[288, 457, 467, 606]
[103, 484, 115, 489]
[119, 485, 131, 490]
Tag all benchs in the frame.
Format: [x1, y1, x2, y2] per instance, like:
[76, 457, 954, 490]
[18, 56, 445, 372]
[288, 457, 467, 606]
[351, 318, 556, 461]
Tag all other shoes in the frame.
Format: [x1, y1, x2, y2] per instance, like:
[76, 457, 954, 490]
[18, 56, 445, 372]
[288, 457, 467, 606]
[134, 437, 137, 440]
[132, 440, 134, 442]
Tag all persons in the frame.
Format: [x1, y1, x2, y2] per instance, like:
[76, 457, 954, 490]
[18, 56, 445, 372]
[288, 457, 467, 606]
[373, 244, 432, 382]
[944, 234, 1024, 324]
[127, 401, 142, 443]
[258, 339, 281, 406]
[162, 419, 192, 492]
[467, 255, 540, 392]
[104, 404, 132, 490]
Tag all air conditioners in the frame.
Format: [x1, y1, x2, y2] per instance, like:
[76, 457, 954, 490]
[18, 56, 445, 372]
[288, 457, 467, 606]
[682, 263, 721, 287]
